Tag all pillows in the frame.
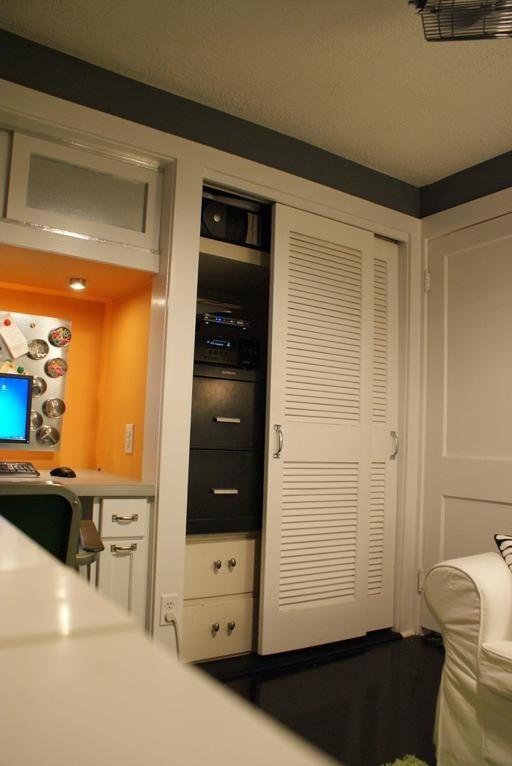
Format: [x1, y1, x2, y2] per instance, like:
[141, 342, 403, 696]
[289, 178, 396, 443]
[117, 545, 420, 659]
[494, 534, 512, 574]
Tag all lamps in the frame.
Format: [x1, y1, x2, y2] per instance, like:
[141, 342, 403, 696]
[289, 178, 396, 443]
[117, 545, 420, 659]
[69, 278, 86, 290]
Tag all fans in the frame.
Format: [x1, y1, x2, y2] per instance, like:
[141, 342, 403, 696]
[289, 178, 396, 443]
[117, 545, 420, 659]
[408, 0, 512, 42]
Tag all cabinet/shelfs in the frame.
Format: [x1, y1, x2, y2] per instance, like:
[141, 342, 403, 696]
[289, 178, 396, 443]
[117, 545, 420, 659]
[0, 469, 155, 632]
[258, 203, 400, 656]
[183, 539, 255, 663]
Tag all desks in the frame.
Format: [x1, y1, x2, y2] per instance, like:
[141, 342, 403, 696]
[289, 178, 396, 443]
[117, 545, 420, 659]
[0, 519, 336, 766]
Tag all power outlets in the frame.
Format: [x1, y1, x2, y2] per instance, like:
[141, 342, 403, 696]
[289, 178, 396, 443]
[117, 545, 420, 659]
[159, 594, 176, 625]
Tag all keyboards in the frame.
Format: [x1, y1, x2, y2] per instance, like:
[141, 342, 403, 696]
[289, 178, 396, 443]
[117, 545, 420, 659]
[0, 461, 40, 478]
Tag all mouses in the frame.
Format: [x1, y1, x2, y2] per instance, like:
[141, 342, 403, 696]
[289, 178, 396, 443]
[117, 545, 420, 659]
[50, 467, 76, 477]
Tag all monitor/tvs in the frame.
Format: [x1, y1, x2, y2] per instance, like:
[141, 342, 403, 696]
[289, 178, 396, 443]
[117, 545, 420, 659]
[0, 372, 33, 444]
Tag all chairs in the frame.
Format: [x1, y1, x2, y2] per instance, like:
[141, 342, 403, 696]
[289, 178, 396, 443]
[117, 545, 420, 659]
[0, 481, 105, 570]
[422, 552, 512, 766]
